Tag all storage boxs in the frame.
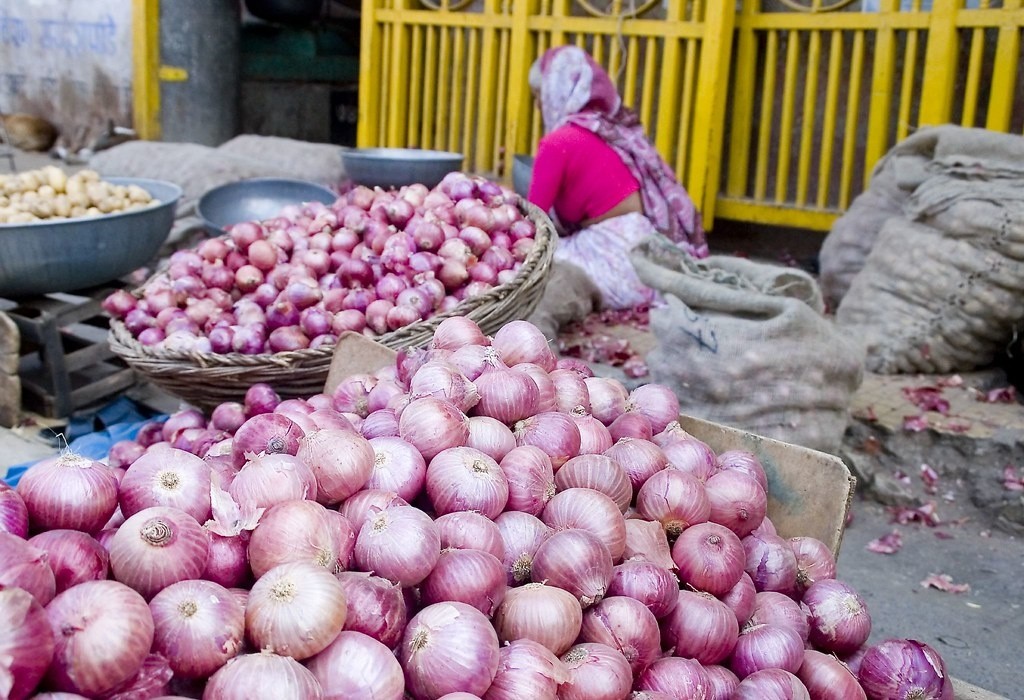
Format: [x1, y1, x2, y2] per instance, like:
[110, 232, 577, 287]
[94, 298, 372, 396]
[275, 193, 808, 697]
[0, 275, 138, 420]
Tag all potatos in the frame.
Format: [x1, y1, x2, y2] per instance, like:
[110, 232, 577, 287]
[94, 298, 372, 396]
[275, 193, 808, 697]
[0, 166, 160, 225]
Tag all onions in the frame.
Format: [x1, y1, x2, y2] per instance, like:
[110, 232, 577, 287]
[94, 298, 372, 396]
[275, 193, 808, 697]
[104, 170, 539, 354]
[0, 315, 957, 700]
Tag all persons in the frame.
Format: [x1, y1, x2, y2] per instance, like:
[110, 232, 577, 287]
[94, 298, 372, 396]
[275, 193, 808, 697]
[524, 44, 692, 310]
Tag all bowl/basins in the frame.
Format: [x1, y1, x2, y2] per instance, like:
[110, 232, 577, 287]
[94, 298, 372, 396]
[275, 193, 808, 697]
[338, 146, 465, 189]
[195, 177, 338, 237]
[513, 154, 534, 199]
[0, 176, 183, 295]
